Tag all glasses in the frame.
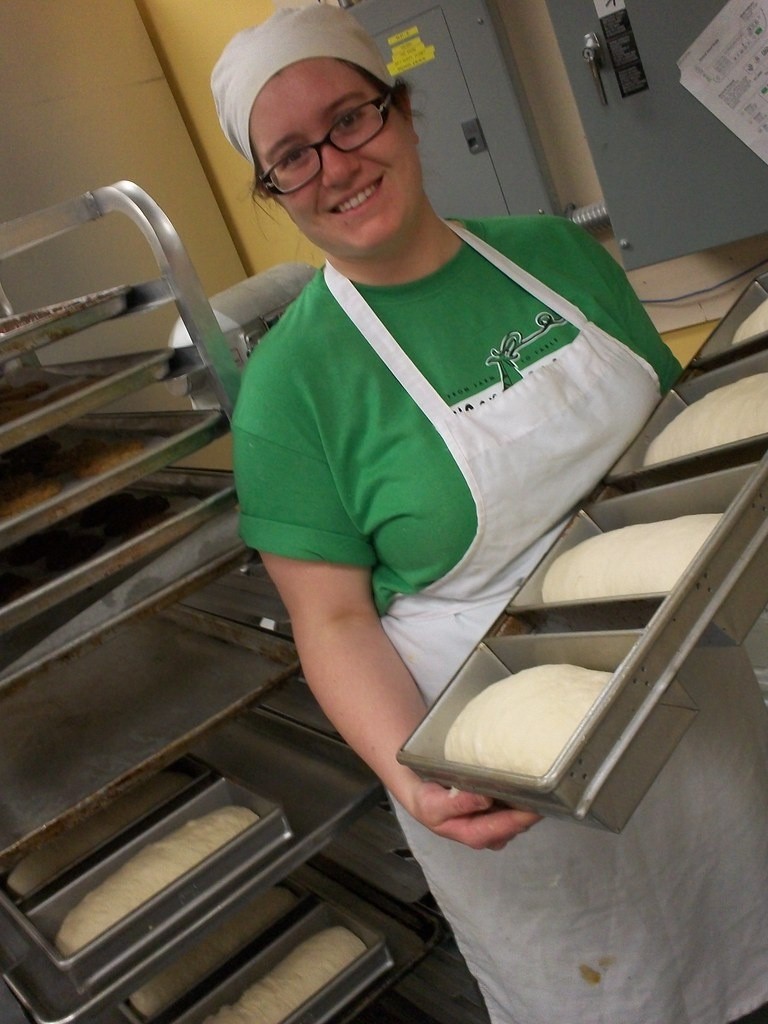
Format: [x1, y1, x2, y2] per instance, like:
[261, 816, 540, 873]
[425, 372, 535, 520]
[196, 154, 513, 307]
[257, 86, 393, 195]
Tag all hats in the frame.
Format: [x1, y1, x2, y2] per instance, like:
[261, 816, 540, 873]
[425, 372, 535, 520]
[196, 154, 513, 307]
[210, 2, 396, 169]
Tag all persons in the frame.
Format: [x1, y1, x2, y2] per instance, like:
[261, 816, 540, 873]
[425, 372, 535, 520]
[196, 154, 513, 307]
[211, 0, 767, 1024]
